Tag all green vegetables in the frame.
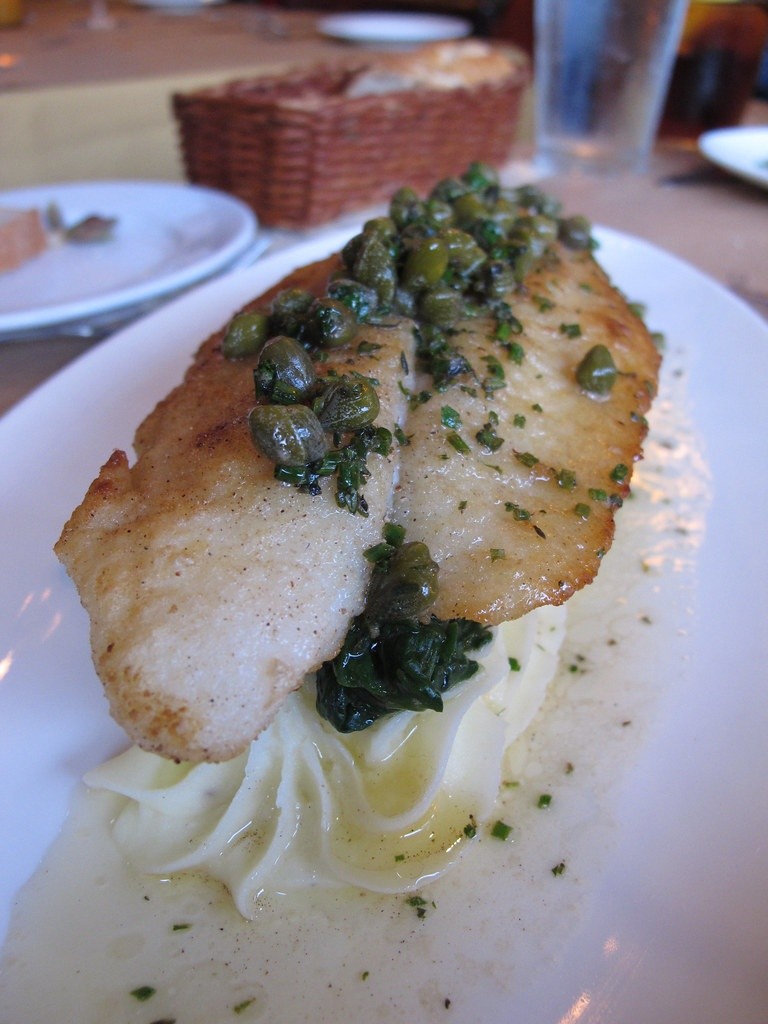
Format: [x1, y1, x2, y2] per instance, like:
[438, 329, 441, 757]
[220, 165, 623, 731]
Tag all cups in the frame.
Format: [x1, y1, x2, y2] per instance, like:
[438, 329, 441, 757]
[533, 0, 689, 183]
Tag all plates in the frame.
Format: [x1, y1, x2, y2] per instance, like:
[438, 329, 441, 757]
[2, 180, 259, 341]
[316, 13, 467, 45]
[2, 203, 767, 1024]
[698, 122, 768, 192]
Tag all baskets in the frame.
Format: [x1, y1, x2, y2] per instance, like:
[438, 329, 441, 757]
[174, 52, 521, 231]
